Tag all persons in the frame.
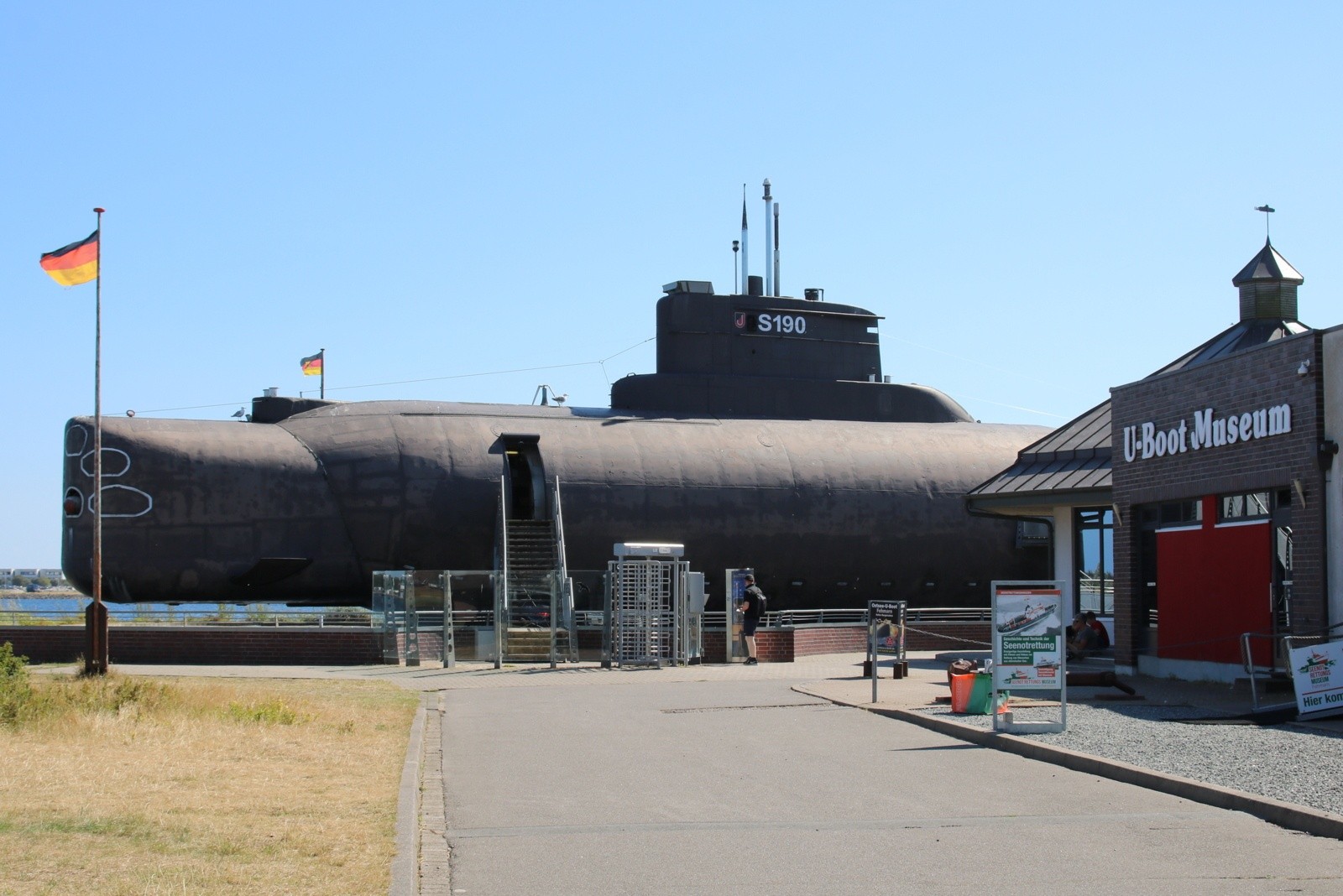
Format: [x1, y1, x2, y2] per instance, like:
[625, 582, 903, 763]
[1066, 613, 1097, 659]
[1087, 610, 1111, 649]
[739, 575, 762, 665]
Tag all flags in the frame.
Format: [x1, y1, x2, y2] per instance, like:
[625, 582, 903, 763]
[300, 351, 323, 375]
[41, 230, 100, 289]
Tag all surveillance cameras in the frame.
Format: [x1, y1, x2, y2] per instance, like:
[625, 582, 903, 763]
[1298, 366, 1309, 377]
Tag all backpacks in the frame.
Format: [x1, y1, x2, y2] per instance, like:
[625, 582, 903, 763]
[747, 587, 767, 617]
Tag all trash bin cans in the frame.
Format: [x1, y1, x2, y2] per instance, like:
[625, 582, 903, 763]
[475, 628, 495, 661]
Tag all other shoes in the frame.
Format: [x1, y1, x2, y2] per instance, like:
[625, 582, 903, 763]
[743, 657, 757, 665]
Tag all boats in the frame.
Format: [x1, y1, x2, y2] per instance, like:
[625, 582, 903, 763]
[61, 179, 1084, 617]
[998, 604, 1058, 633]
